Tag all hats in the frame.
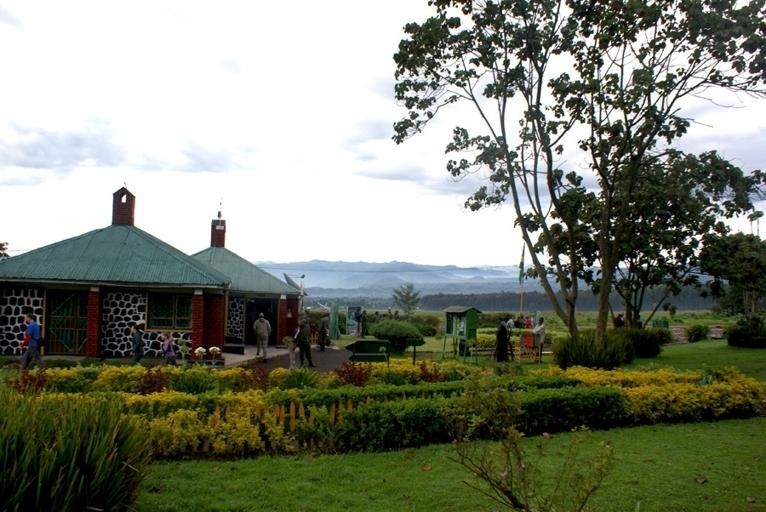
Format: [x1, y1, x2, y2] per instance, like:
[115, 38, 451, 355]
[259, 312, 264, 317]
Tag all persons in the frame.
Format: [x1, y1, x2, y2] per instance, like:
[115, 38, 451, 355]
[354, 306, 401, 339]
[252, 312, 271, 357]
[613, 313, 625, 328]
[18, 312, 48, 370]
[637, 315, 642, 328]
[495, 312, 545, 363]
[292, 323, 316, 367]
[154, 331, 177, 365]
[291, 309, 330, 352]
[125, 324, 145, 365]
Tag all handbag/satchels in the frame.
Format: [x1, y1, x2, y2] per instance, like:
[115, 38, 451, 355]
[36, 337, 44, 346]
